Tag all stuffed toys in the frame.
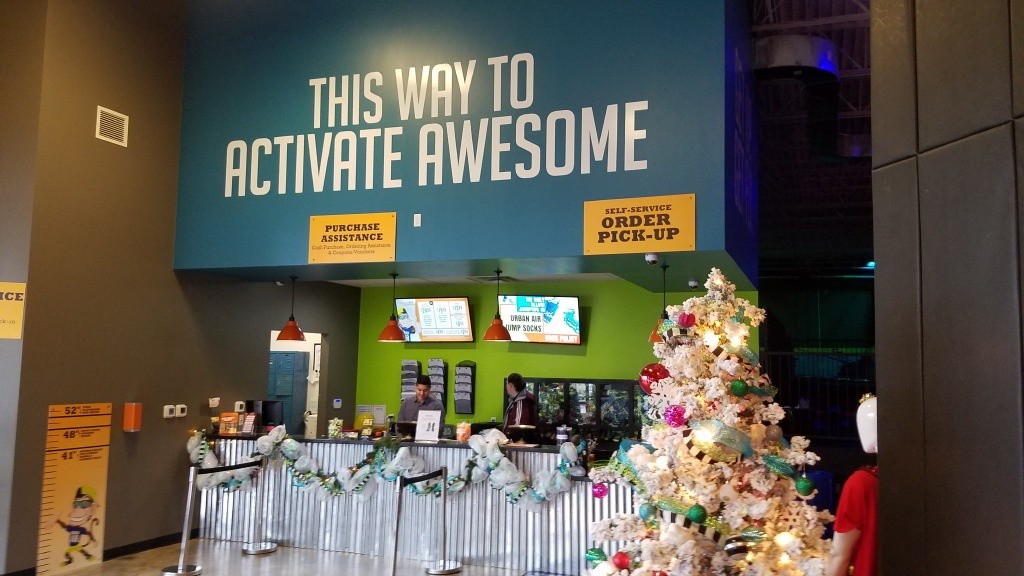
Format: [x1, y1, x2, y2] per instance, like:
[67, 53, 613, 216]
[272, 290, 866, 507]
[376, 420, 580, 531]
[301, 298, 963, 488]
[589, 295, 820, 576]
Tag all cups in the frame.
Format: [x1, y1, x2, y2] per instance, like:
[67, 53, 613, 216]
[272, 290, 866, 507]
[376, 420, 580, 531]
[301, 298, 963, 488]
[555, 424, 571, 445]
[456, 421, 471, 443]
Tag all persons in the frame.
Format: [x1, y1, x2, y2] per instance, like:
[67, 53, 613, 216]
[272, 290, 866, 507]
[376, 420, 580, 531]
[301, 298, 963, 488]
[823, 397, 877, 576]
[503, 372, 537, 440]
[397, 374, 446, 438]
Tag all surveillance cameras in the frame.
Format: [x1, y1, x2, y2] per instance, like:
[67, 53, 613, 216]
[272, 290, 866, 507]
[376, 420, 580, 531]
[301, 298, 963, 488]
[644, 253, 660, 266]
[687, 280, 699, 290]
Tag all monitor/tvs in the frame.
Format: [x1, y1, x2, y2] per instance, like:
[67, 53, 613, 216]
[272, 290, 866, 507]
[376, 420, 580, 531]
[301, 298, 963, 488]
[506, 425, 539, 444]
[470, 421, 503, 437]
[396, 421, 417, 439]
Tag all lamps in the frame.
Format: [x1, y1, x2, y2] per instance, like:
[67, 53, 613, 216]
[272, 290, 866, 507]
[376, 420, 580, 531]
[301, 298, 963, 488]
[377, 272, 405, 343]
[647, 266, 668, 342]
[481, 270, 511, 342]
[275, 276, 308, 342]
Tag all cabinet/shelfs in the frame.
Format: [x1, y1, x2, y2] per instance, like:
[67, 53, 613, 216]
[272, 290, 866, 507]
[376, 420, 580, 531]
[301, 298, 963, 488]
[503, 376, 654, 459]
[268, 351, 311, 438]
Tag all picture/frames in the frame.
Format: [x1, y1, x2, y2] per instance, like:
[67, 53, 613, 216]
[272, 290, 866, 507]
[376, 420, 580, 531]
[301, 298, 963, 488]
[313, 343, 320, 372]
